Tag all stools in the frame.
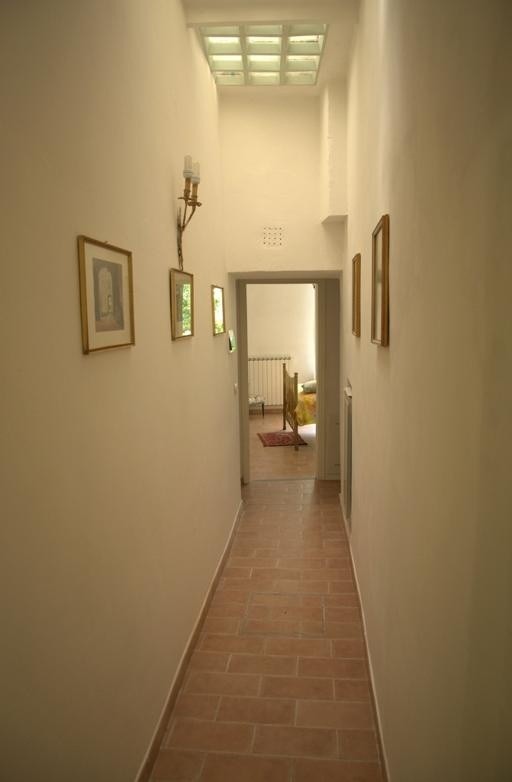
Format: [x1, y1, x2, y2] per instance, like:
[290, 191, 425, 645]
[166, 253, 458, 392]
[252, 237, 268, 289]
[248, 394, 264, 417]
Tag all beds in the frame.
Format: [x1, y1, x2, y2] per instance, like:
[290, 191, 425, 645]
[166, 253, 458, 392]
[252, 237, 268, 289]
[283, 362, 317, 452]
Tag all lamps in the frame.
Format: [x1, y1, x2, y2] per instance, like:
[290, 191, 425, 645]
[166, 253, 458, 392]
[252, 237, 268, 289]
[177, 154, 202, 231]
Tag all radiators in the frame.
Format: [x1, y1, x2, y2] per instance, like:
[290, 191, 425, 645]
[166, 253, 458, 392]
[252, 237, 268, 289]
[247, 353, 293, 406]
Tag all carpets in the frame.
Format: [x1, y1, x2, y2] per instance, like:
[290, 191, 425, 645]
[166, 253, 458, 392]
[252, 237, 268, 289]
[257, 430, 308, 447]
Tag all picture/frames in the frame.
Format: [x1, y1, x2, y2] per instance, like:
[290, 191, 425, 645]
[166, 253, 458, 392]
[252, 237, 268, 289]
[351, 254, 361, 336]
[371, 215, 388, 346]
[211, 284, 226, 335]
[76, 234, 135, 355]
[170, 268, 194, 339]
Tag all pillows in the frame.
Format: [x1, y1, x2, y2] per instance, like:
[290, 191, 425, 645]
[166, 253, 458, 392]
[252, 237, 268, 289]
[301, 380, 316, 393]
